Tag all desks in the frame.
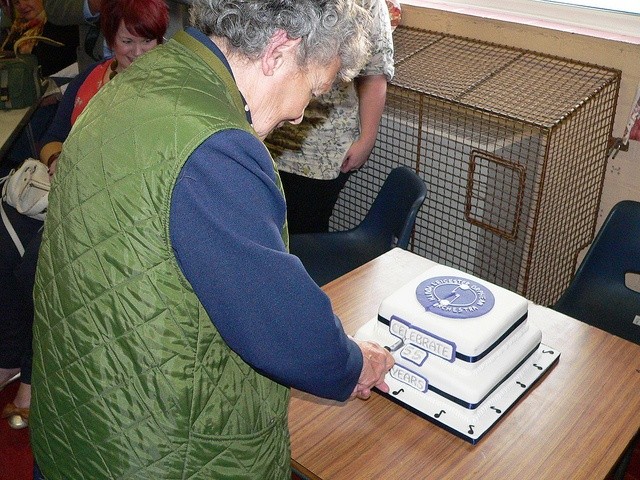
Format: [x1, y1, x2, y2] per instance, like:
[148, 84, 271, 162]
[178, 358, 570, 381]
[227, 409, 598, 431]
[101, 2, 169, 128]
[263, 244, 639, 480]
[0, 77, 51, 163]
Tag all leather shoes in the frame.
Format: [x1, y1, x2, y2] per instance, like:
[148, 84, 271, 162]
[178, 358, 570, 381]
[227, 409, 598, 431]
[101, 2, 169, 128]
[2, 400, 30, 430]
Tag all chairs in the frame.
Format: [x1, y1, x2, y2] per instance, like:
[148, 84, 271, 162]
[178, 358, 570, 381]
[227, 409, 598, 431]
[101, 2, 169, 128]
[271, 163, 429, 289]
[548, 199, 640, 347]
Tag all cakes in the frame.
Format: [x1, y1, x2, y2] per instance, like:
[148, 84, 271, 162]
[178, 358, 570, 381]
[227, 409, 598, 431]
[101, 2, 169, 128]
[353, 267, 542, 412]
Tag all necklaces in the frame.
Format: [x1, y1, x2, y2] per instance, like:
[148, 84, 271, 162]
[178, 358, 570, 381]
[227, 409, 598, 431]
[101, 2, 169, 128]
[109, 61, 118, 80]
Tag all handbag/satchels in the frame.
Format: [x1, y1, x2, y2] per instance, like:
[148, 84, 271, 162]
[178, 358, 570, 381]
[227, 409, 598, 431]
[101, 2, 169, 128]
[1, 36, 64, 111]
[0, 157, 49, 257]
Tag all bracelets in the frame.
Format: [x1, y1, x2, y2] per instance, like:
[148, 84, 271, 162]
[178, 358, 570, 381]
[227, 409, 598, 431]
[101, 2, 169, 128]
[46, 150, 61, 169]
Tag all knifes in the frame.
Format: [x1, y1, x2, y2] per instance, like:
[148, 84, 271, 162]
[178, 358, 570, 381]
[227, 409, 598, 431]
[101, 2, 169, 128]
[384, 340, 405, 352]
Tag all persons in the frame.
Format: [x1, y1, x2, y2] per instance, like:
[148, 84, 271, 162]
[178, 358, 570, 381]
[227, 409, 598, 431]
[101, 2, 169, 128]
[43, 0, 103, 75]
[0, 1, 80, 110]
[262, 0, 395, 235]
[3, 0, 169, 430]
[29, 0, 397, 479]
